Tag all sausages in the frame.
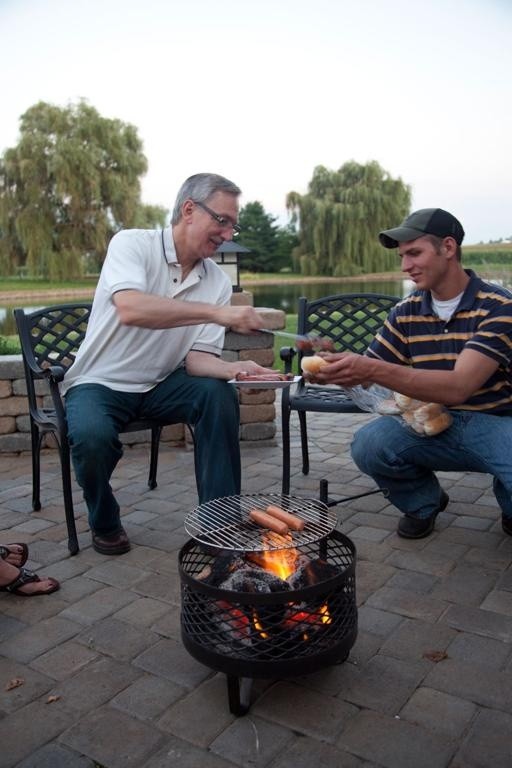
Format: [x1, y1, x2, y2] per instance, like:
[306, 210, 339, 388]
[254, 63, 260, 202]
[236, 371, 294, 382]
[294, 339, 333, 351]
[248, 506, 305, 536]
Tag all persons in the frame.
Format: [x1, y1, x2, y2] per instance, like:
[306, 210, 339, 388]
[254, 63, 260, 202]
[0, 543, 60, 597]
[62, 172, 280, 556]
[302, 207, 511, 539]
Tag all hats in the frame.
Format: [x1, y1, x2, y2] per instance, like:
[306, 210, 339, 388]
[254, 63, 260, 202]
[378, 208, 464, 249]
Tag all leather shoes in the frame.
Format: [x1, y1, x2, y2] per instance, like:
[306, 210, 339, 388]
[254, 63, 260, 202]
[91, 526, 131, 555]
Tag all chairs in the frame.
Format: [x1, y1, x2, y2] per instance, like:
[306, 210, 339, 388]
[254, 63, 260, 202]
[279, 292, 412, 509]
[14, 295, 240, 556]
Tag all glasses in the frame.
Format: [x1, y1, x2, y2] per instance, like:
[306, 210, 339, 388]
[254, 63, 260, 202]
[192, 199, 240, 236]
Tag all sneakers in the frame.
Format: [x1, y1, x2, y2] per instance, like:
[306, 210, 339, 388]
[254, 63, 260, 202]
[502, 512, 512, 536]
[397, 487, 449, 539]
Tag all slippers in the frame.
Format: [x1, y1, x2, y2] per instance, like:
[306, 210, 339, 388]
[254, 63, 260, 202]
[0, 542, 60, 595]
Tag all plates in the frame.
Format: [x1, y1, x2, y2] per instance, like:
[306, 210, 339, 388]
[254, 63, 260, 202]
[227, 375, 303, 390]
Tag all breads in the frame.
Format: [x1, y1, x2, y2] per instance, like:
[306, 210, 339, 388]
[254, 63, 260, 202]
[374, 390, 453, 438]
[299, 355, 331, 376]
